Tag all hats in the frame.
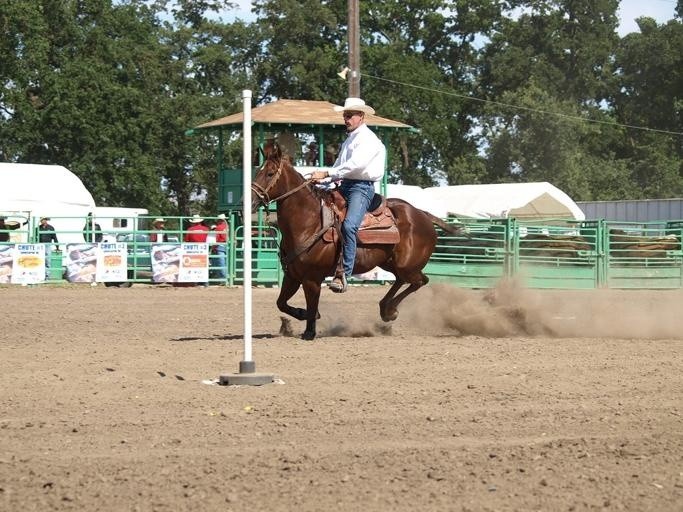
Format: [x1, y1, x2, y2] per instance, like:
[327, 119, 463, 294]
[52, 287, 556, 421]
[152, 218, 167, 225]
[333, 97, 376, 115]
[215, 213, 226, 223]
[188, 214, 204, 223]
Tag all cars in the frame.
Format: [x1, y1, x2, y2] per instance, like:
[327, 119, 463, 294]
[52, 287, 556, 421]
[106, 233, 177, 286]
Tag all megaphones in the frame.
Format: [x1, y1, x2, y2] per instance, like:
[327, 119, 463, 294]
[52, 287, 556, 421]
[337, 66, 349, 80]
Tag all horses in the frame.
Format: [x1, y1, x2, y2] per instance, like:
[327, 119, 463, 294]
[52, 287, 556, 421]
[435, 218, 681, 266]
[237, 140, 473, 341]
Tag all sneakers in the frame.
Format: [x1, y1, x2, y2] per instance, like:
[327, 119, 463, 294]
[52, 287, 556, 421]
[329, 278, 348, 293]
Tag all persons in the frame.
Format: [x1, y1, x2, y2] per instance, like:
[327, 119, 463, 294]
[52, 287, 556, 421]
[0, 215, 29, 242]
[35, 216, 58, 250]
[149, 218, 168, 242]
[184, 214, 209, 245]
[212, 213, 229, 284]
[267, 222, 275, 247]
[312, 97, 386, 290]
[210, 225, 218, 255]
[83, 212, 102, 243]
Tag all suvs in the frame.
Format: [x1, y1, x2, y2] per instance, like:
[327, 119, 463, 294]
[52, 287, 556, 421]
[582, 217, 605, 246]
[662, 219, 683, 238]
